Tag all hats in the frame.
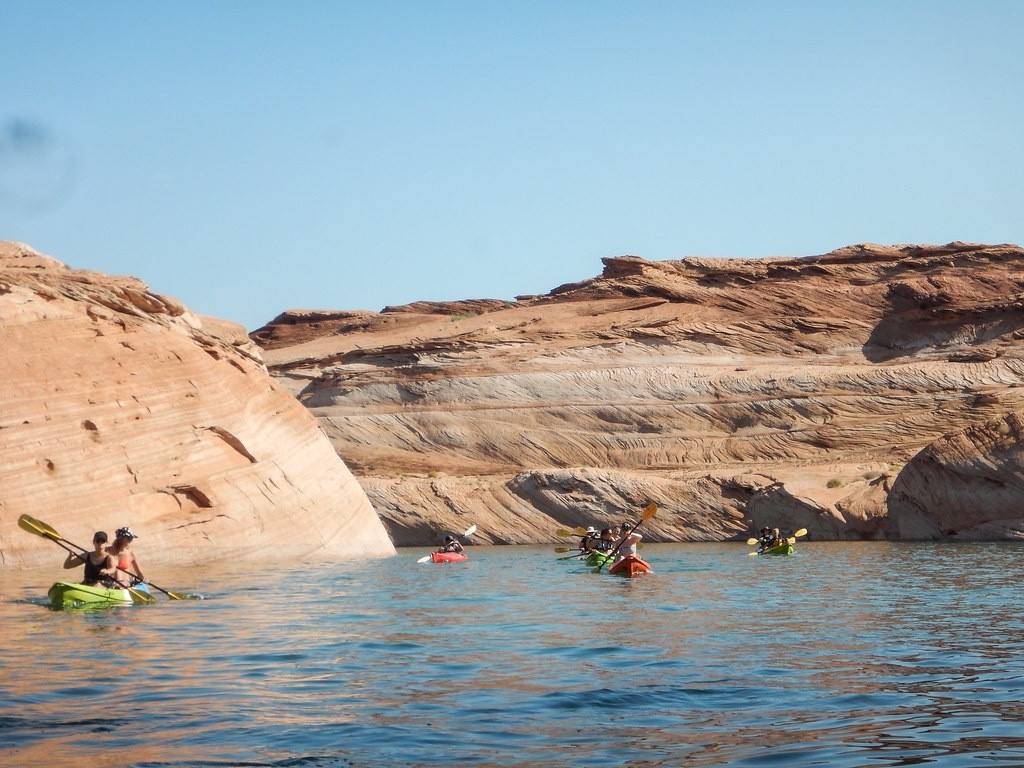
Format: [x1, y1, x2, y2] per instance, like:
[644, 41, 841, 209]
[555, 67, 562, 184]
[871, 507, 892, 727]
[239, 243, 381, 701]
[586, 526, 595, 533]
[445, 535, 454, 541]
[115, 526, 138, 538]
[760, 526, 772, 534]
[622, 522, 631, 530]
[94, 531, 107, 541]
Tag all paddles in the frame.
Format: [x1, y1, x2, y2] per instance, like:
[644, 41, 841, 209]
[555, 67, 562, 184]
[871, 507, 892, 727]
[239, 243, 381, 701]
[37, 519, 191, 601]
[554, 526, 643, 561]
[592, 502, 658, 572]
[416, 525, 477, 563]
[747, 537, 795, 545]
[747, 528, 808, 555]
[17, 514, 156, 603]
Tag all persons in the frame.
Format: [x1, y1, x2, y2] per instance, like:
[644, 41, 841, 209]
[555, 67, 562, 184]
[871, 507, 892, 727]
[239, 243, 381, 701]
[587, 528, 619, 554]
[438, 535, 464, 552]
[612, 522, 643, 560]
[103, 527, 149, 589]
[578, 526, 597, 552]
[62, 531, 115, 590]
[759, 526, 774, 549]
[762, 528, 788, 549]
[610, 526, 620, 543]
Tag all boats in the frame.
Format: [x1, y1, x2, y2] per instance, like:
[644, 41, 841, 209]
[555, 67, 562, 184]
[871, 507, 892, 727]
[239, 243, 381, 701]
[586, 550, 615, 566]
[763, 542, 793, 556]
[431, 551, 468, 563]
[609, 555, 650, 575]
[47, 579, 149, 609]
